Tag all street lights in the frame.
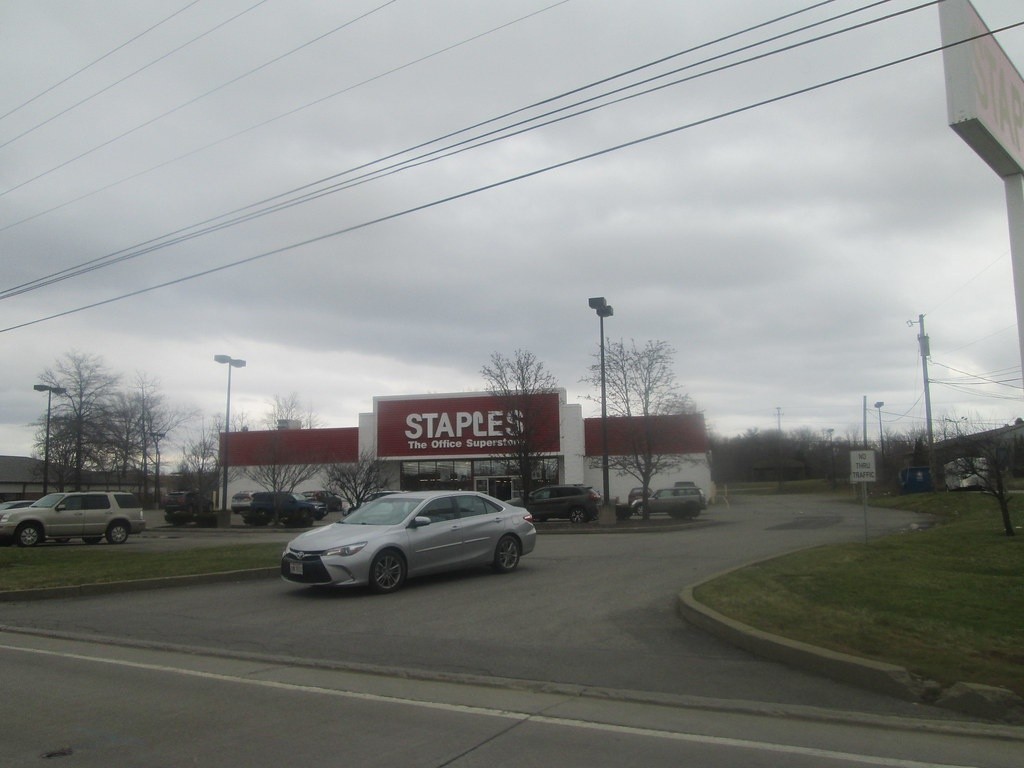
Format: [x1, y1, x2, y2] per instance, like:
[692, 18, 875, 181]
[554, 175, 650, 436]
[34, 385, 66, 495]
[588, 297, 613, 526]
[214, 355, 246, 512]
[874, 402, 885, 464]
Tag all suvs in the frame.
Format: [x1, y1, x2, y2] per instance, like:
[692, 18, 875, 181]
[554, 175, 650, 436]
[164, 491, 213, 515]
[632, 487, 708, 519]
[251, 491, 328, 522]
[231, 491, 254, 514]
[505, 484, 602, 523]
[0, 491, 146, 547]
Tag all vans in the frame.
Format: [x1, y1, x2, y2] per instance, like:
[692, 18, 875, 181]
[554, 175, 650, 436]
[674, 481, 695, 488]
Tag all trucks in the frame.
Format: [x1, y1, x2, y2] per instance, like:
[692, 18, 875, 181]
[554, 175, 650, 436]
[944, 458, 987, 493]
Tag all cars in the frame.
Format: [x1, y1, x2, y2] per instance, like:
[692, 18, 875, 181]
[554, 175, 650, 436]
[280, 490, 536, 594]
[300, 491, 350, 512]
[343, 491, 410, 517]
[628, 487, 654, 508]
[0, 501, 35, 510]
